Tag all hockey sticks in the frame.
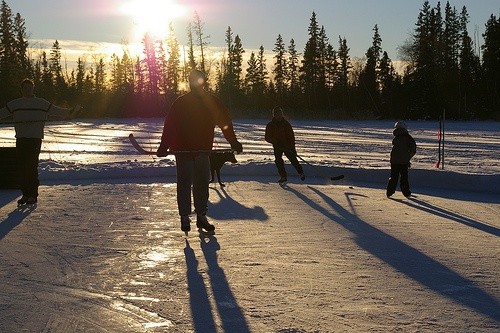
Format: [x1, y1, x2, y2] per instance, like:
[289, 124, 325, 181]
[285, 146, 344, 180]
[129, 133, 242, 156]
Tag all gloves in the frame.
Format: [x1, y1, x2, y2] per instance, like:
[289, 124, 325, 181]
[157, 142, 168, 157]
[230, 138, 243, 154]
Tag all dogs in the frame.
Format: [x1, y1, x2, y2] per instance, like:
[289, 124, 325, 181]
[209, 151, 237, 187]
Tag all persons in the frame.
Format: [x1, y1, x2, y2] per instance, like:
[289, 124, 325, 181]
[0, 78, 76, 204]
[156, 70, 243, 231]
[386, 121, 416, 198]
[264, 107, 305, 183]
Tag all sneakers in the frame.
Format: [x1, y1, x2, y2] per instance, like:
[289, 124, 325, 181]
[181, 216, 190, 236]
[278, 179, 287, 185]
[17, 196, 27, 206]
[27, 197, 37, 208]
[196, 216, 215, 234]
[300, 173, 305, 181]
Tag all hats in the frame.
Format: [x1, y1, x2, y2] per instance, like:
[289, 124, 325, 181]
[273, 104, 283, 118]
[394, 121, 407, 129]
